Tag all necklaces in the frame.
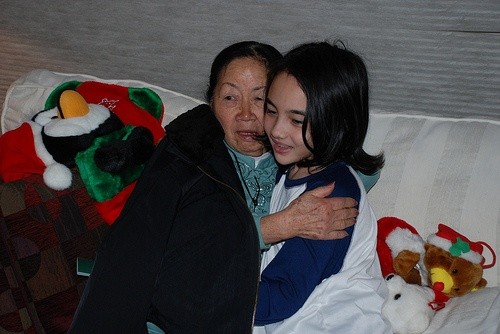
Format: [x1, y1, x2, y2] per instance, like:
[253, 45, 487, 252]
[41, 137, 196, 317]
[228, 148, 261, 207]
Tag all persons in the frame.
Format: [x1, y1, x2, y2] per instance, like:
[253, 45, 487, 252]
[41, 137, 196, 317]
[69, 41, 387, 334]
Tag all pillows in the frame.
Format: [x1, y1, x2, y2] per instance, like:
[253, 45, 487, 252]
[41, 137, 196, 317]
[0, 166, 110, 333]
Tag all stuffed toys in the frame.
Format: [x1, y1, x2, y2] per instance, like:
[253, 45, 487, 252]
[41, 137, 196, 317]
[376, 217, 497, 334]
[0, 80, 167, 226]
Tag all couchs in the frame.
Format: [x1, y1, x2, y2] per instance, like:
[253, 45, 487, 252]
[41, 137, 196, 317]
[2, 69, 500, 334]
